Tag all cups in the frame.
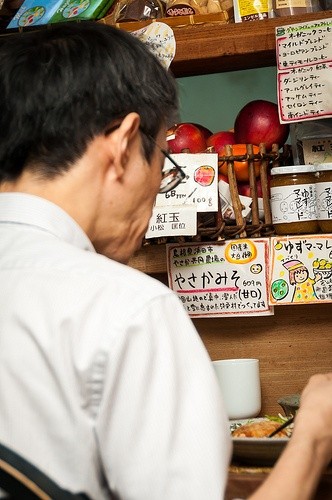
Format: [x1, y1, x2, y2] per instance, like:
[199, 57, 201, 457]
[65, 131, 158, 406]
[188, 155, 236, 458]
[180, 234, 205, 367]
[212, 359, 262, 420]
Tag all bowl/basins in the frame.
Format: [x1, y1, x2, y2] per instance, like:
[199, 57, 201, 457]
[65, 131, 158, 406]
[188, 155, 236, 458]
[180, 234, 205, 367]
[227, 416, 291, 461]
[277, 396, 300, 418]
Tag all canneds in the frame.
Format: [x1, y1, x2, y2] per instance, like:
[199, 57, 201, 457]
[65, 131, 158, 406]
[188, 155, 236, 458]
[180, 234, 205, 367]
[269, 162, 332, 235]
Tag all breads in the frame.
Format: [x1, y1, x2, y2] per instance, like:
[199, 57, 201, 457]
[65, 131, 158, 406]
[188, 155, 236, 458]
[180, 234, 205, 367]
[163, 0, 233, 17]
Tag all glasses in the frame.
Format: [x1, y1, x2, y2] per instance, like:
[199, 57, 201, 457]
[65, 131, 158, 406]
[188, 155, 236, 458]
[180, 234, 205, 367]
[106, 126, 186, 194]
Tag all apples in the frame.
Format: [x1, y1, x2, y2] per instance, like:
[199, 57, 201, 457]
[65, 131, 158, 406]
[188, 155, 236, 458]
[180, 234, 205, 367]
[164, 100, 291, 198]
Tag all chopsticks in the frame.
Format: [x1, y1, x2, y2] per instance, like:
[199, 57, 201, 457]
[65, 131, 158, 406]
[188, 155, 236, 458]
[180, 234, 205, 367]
[268, 416, 294, 438]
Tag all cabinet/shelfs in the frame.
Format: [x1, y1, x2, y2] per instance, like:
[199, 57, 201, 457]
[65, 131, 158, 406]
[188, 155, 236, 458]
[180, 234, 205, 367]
[127, 9, 332, 271]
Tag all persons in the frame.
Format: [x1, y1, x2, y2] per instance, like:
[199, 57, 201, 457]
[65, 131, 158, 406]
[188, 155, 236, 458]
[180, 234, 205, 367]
[0, 20, 332, 500]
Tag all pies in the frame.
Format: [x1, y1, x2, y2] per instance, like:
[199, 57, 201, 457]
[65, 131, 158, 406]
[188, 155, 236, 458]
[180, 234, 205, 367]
[231, 421, 289, 439]
